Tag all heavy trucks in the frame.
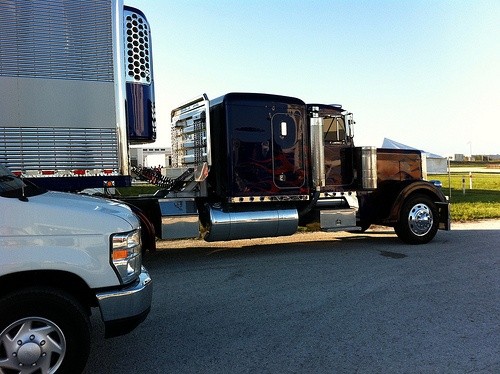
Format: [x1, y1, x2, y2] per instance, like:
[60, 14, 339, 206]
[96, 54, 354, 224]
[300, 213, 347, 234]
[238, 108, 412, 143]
[0, 0, 450, 244]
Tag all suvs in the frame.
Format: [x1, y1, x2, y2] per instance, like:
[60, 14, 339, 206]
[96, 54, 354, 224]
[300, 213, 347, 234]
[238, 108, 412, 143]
[0, 162, 153, 374]
